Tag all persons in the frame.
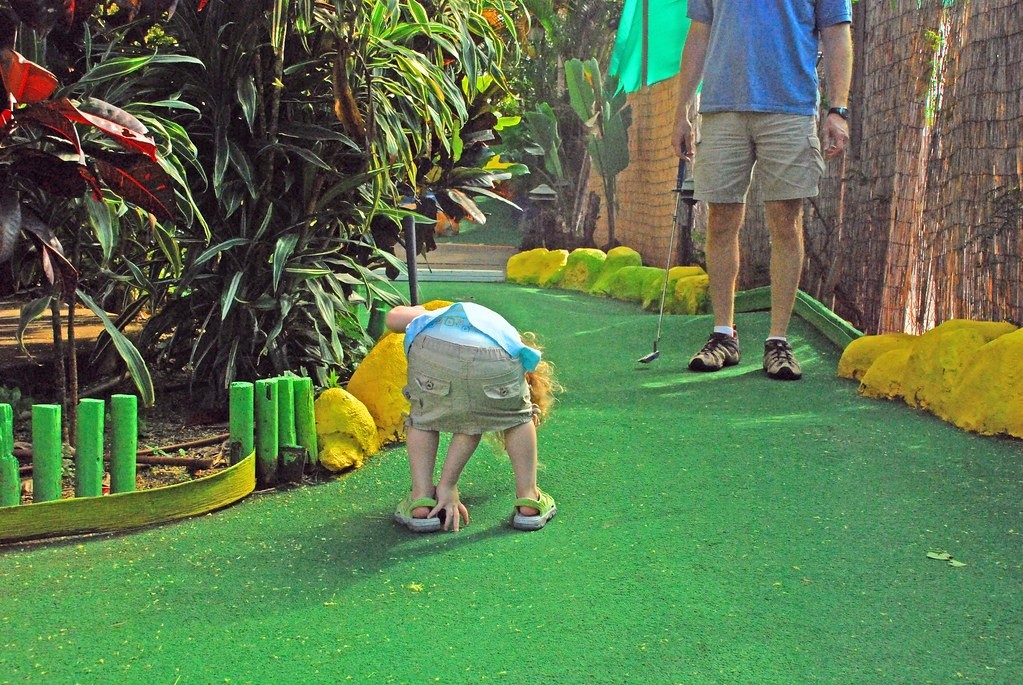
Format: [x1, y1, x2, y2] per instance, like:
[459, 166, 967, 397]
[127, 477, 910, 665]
[671, 0, 852, 380]
[385, 302, 564, 532]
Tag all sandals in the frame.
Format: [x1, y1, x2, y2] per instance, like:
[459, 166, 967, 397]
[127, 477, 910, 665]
[394, 488, 441, 532]
[513, 486, 556, 531]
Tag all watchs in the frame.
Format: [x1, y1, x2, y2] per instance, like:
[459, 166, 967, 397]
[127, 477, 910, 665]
[827, 107, 850, 120]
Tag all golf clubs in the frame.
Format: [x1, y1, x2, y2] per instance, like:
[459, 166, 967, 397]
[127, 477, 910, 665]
[637, 141, 687, 363]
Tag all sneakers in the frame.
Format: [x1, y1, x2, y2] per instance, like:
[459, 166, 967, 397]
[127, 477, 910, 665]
[688, 324, 740, 372]
[764, 339, 801, 380]
